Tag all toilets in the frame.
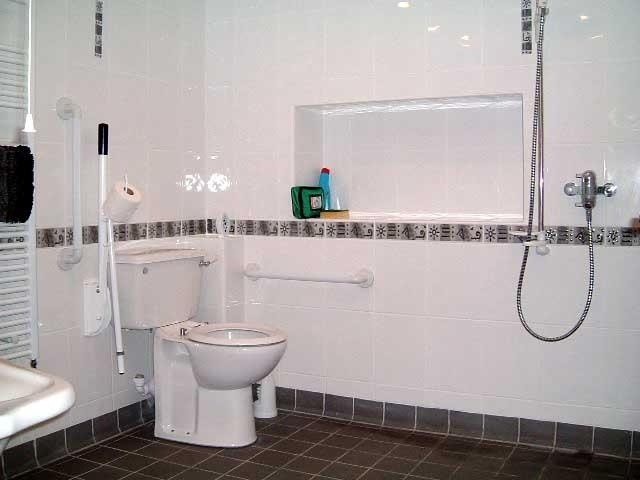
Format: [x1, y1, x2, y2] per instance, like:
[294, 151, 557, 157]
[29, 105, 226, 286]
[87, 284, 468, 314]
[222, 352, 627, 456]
[107, 250, 287, 449]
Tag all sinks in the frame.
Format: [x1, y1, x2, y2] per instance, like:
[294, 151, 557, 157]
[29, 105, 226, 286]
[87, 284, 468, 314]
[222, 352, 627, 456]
[1, 357, 76, 441]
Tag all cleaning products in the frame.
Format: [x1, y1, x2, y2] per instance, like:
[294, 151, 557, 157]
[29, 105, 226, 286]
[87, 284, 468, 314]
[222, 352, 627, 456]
[318, 168, 331, 210]
